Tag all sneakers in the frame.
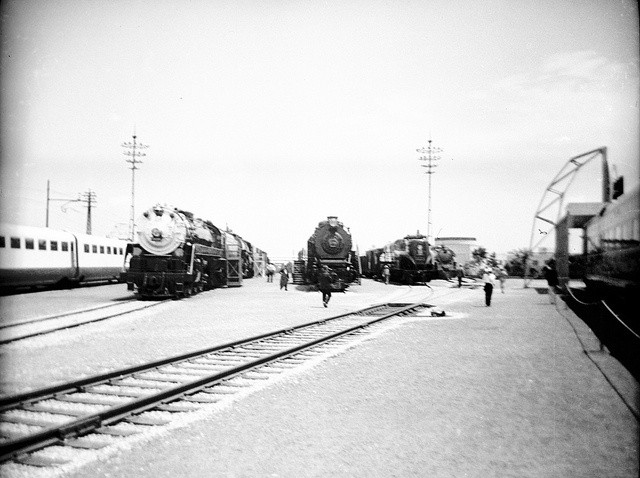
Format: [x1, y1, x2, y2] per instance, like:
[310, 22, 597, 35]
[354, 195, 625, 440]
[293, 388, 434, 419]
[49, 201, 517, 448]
[323, 302, 327, 307]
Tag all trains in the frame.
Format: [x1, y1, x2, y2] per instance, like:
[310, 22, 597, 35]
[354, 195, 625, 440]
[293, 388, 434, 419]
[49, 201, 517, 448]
[305, 215, 362, 293]
[0, 224, 131, 289]
[129, 204, 271, 299]
[580, 182, 639, 377]
[432, 246, 464, 278]
[366, 231, 438, 284]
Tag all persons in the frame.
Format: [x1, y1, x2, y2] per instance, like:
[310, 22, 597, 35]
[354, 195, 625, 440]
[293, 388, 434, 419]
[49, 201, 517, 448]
[482, 267, 495, 308]
[543, 257, 559, 305]
[320, 265, 337, 307]
[456, 264, 465, 288]
[279, 265, 289, 291]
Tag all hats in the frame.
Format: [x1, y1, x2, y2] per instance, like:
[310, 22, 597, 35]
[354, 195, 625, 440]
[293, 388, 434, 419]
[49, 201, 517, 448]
[485, 268, 493, 273]
